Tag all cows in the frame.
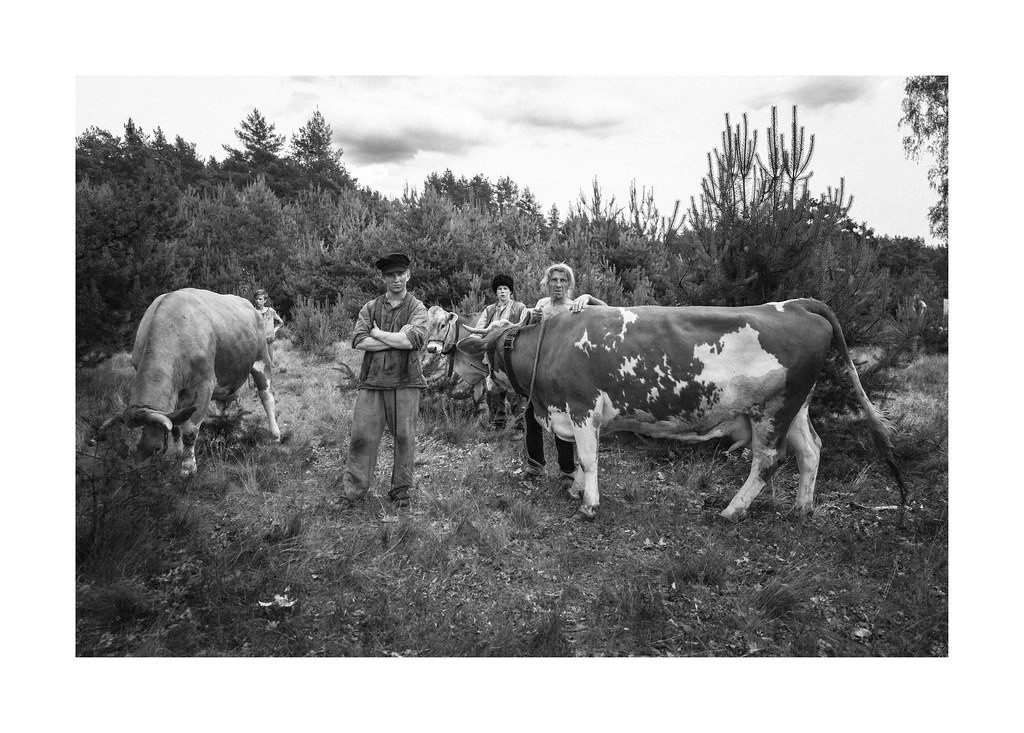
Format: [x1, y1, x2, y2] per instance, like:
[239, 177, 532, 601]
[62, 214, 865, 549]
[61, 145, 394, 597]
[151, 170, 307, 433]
[86, 288, 282, 480]
[456, 297, 906, 526]
[427, 305, 484, 428]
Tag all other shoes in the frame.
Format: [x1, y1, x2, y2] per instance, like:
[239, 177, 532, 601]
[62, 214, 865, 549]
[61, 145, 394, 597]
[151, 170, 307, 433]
[395, 497, 409, 507]
[332, 498, 352, 511]
[510, 429, 524, 441]
[514, 466, 548, 480]
[560, 475, 574, 482]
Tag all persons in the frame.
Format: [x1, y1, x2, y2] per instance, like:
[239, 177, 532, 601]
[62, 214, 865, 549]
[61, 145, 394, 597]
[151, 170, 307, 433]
[254, 290, 283, 364]
[473, 274, 527, 434]
[522, 264, 608, 501]
[331, 253, 429, 512]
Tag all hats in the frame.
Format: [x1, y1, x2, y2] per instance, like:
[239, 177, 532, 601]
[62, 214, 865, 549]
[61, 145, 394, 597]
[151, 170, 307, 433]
[492, 274, 514, 296]
[375, 253, 410, 276]
